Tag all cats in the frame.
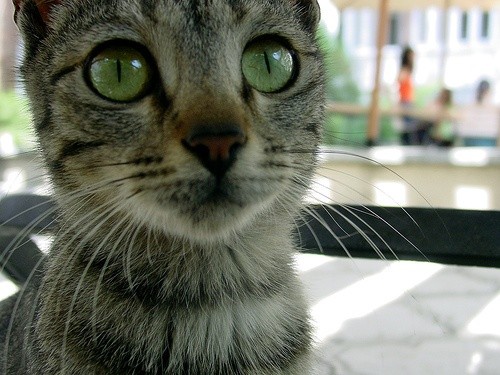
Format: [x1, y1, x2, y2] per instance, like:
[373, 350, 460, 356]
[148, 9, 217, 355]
[1, 0, 435, 374]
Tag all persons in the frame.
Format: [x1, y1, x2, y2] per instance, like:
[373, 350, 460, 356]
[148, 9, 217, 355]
[395, 42, 499, 148]
